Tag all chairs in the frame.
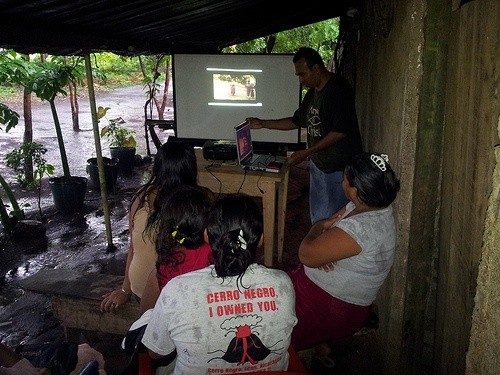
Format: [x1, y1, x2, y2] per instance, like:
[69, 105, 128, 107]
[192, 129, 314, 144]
[139, 345, 309, 375]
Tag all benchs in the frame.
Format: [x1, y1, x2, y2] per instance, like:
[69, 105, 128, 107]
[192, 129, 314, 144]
[19, 266, 145, 336]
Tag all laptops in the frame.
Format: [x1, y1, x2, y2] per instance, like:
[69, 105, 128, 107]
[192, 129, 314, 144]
[234, 121, 286, 172]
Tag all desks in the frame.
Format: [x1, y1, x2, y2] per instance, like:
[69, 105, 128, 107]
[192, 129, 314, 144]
[148, 148, 291, 268]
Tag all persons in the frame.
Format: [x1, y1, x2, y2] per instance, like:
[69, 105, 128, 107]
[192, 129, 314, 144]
[288, 152, 400, 349]
[141, 194, 298, 375]
[246, 46, 365, 227]
[0, 343, 37, 375]
[101, 141, 215, 316]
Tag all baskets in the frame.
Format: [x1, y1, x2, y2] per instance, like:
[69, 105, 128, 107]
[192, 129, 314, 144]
[48, 175, 88, 213]
[87, 157, 115, 191]
[111, 147, 135, 174]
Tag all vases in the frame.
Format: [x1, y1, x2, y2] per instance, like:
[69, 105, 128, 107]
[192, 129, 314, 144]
[87, 157, 120, 193]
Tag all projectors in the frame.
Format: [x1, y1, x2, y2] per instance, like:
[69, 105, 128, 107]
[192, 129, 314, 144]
[203, 140, 238, 159]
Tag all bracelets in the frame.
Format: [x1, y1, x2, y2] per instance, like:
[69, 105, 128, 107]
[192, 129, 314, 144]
[121, 288, 132, 295]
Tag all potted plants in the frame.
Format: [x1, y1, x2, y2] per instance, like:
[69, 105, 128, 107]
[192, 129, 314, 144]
[6, 142, 56, 246]
[101, 118, 137, 173]
[0, 47, 91, 217]
